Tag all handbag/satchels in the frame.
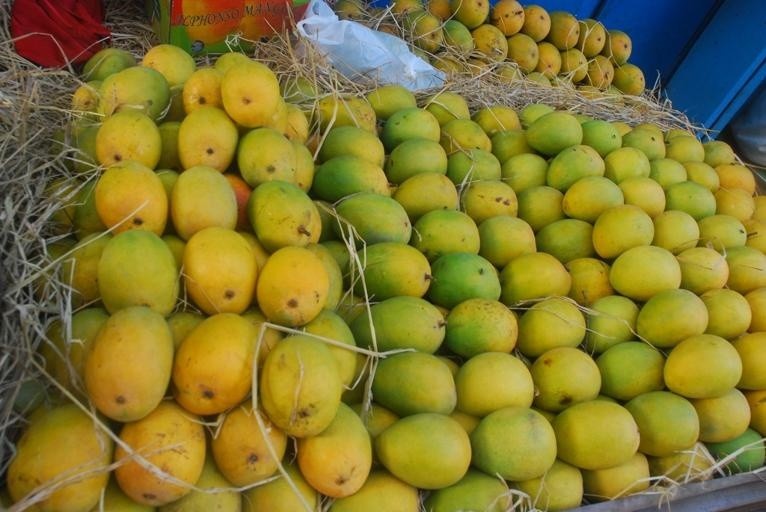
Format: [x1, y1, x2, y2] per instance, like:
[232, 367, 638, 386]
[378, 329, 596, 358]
[13, 0, 112, 70]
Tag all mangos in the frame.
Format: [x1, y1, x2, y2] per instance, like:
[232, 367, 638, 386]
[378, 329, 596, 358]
[1, 0, 766, 511]
